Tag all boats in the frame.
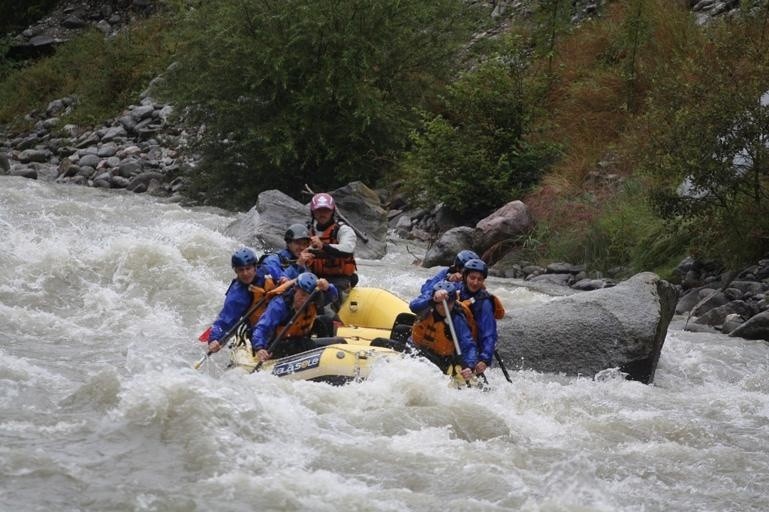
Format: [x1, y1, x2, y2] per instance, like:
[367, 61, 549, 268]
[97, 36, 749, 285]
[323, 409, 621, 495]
[230, 284, 480, 388]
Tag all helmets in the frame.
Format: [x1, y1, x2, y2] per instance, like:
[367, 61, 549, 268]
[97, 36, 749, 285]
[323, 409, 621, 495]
[310, 193, 335, 211]
[232, 248, 259, 268]
[432, 280, 458, 303]
[454, 250, 487, 279]
[293, 272, 320, 297]
[285, 224, 313, 242]
